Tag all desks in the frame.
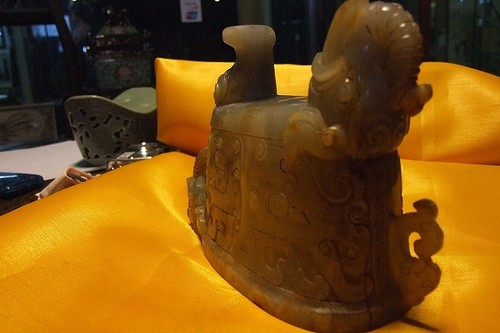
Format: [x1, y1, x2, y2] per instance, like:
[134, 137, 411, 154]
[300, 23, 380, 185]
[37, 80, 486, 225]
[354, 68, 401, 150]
[0, 141, 151, 184]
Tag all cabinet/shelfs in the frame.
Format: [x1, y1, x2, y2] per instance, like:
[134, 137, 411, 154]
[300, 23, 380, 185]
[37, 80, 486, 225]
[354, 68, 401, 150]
[0, 103, 58, 153]
